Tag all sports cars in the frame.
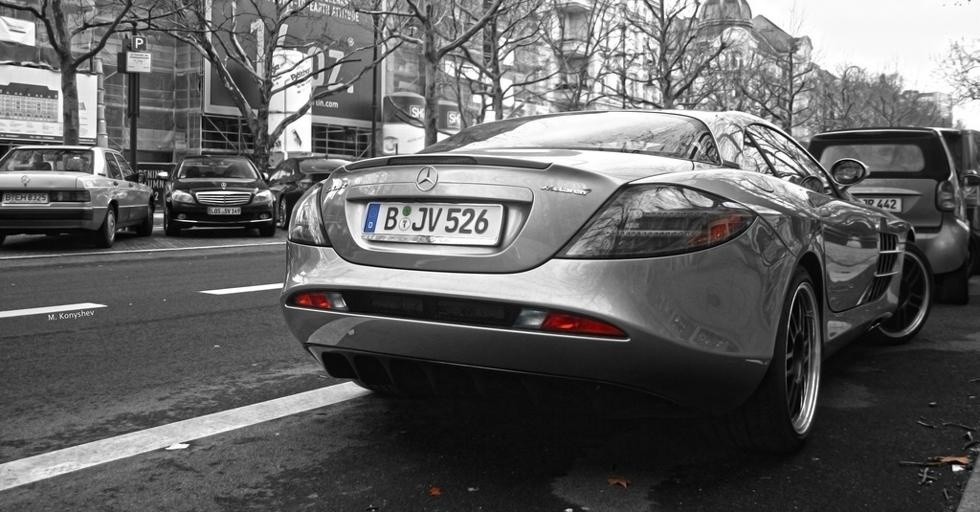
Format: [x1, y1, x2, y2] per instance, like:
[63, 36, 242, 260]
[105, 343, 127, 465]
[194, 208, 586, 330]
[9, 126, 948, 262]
[282, 107, 940, 454]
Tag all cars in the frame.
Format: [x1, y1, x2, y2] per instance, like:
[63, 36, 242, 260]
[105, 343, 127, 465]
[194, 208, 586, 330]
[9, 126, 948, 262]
[263, 156, 351, 231]
[0, 145, 156, 249]
[800, 127, 974, 306]
[157, 153, 279, 240]
[940, 127, 980, 281]
